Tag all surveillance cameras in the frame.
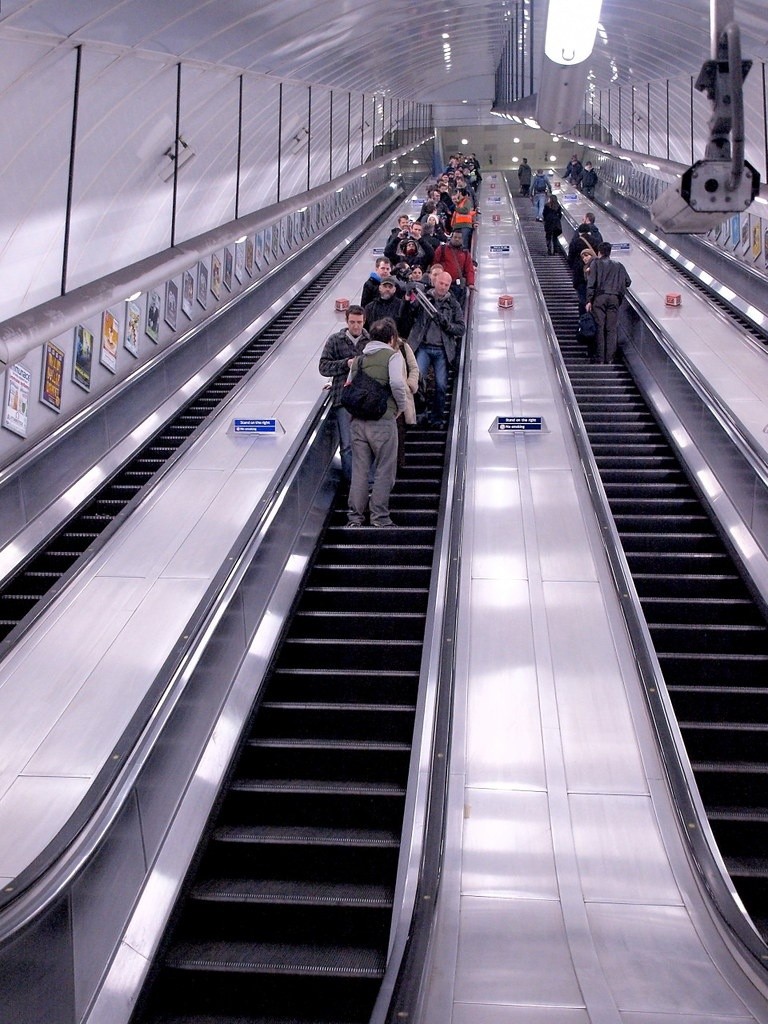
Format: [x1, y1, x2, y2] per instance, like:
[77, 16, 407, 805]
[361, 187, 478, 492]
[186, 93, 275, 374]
[649, 159, 762, 234]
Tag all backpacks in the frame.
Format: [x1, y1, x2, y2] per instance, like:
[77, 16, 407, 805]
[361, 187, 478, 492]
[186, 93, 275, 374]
[534, 179, 546, 191]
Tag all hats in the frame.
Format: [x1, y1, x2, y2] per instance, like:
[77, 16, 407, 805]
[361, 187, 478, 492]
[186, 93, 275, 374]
[468, 162, 475, 167]
[427, 214, 439, 224]
[447, 170, 455, 175]
[381, 277, 395, 286]
[580, 249, 595, 257]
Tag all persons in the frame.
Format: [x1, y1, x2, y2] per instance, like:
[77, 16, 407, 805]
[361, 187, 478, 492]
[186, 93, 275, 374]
[518, 153, 632, 365]
[319, 153, 481, 526]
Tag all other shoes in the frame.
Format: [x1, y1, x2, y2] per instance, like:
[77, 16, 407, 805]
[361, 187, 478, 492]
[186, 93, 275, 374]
[593, 361, 604, 365]
[607, 360, 613, 364]
[536, 217, 543, 222]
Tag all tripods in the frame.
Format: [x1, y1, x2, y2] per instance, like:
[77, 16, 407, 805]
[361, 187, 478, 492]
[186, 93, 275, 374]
[412, 287, 443, 326]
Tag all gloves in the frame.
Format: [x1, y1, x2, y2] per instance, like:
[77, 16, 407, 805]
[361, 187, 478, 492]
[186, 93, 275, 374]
[432, 311, 448, 330]
[406, 283, 418, 296]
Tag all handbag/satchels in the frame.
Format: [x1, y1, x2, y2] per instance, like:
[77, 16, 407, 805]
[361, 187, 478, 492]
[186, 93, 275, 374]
[340, 370, 387, 422]
[576, 310, 596, 345]
[460, 278, 467, 289]
